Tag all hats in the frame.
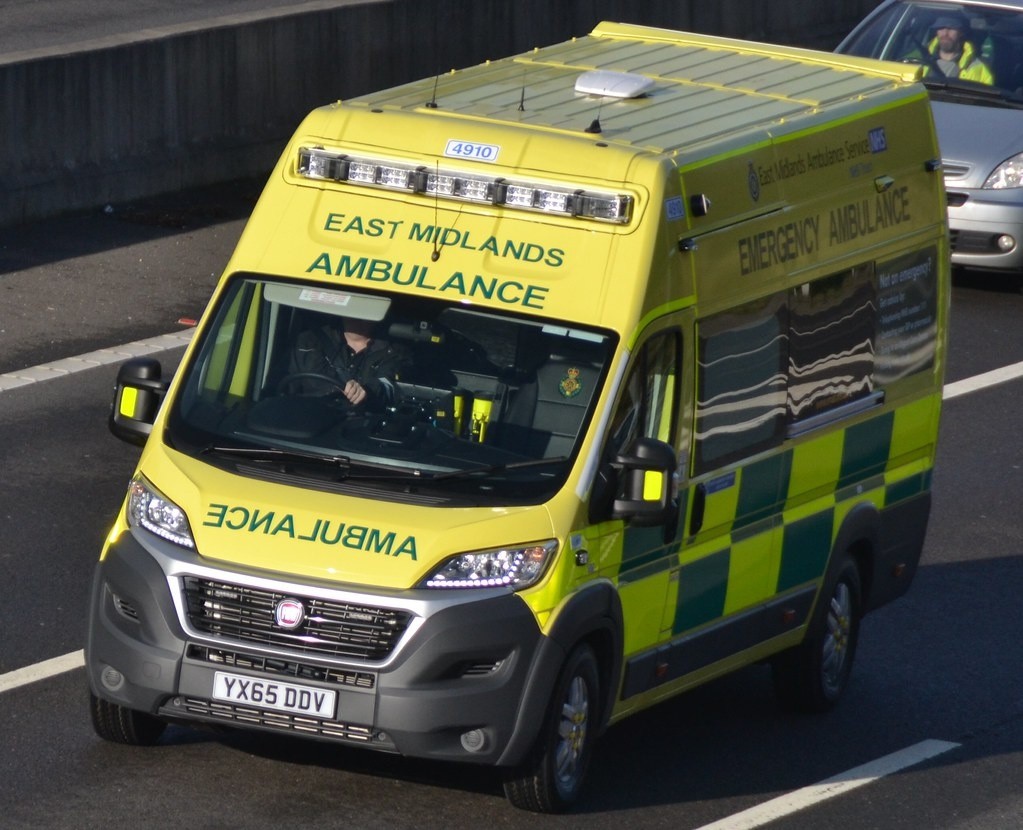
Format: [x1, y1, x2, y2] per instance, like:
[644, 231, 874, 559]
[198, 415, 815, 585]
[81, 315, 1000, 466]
[931, 11, 971, 33]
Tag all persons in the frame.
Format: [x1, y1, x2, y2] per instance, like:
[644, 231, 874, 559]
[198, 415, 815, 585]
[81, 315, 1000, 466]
[899, 10, 994, 85]
[287, 290, 402, 419]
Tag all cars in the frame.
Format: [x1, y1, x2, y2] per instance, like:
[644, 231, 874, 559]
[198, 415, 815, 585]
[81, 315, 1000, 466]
[830, 0, 1023, 277]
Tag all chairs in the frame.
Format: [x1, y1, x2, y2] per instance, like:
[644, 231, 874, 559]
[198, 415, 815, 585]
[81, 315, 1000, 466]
[500, 361, 597, 461]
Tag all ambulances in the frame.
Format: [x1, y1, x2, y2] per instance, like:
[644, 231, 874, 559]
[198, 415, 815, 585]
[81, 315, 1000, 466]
[82, 20, 951, 815]
